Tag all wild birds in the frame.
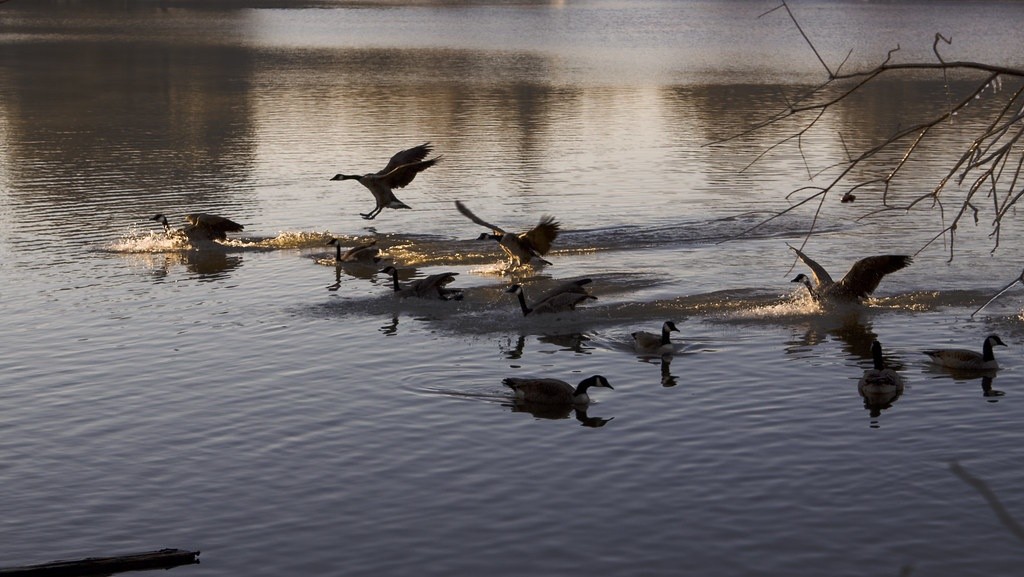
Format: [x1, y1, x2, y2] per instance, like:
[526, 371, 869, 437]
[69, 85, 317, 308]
[922, 334, 1008, 370]
[508, 399, 617, 429]
[455, 199, 563, 273]
[500, 375, 615, 405]
[378, 266, 464, 302]
[330, 141, 446, 220]
[924, 370, 1007, 403]
[325, 238, 394, 265]
[630, 321, 681, 352]
[504, 278, 599, 318]
[149, 213, 245, 243]
[784, 241, 915, 305]
[858, 340, 904, 410]
